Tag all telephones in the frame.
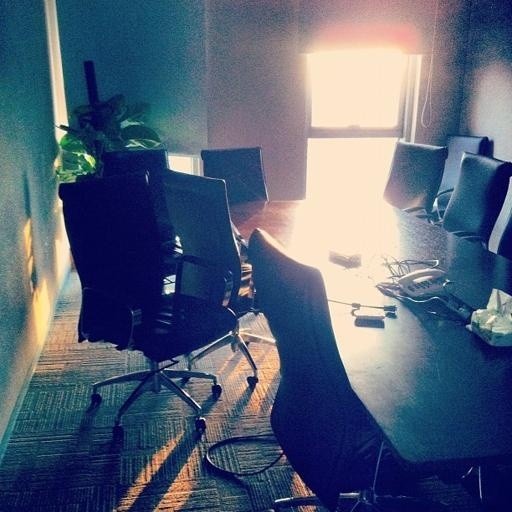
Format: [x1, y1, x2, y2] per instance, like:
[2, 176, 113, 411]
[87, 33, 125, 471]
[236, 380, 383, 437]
[397, 268, 451, 297]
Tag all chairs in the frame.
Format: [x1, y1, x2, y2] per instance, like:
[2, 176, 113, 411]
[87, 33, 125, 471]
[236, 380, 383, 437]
[247, 228, 451, 511]
[384, 142, 448, 221]
[58, 149, 277, 441]
[433, 151, 512, 249]
[434, 134, 490, 221]
[199, 145, 268, 316]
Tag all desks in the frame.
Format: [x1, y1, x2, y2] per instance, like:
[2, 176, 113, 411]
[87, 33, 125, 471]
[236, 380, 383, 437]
[229, 194, 512, 507]
